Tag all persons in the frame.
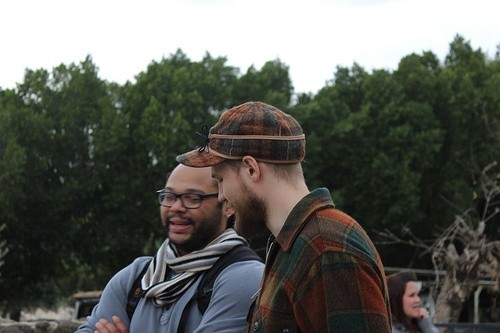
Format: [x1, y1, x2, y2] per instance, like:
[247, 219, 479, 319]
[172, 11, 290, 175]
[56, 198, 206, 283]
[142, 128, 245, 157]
[386, 270, 440, 333]
[76, 164, 266, 333]
[176, 102, 393, 333]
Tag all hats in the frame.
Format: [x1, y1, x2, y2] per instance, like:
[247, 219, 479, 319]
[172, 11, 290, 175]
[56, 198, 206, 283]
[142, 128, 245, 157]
[175, 101, 306, 168]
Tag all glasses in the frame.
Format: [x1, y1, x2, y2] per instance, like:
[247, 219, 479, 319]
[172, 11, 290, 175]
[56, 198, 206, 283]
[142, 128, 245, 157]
[155, 188, 218, 209]
